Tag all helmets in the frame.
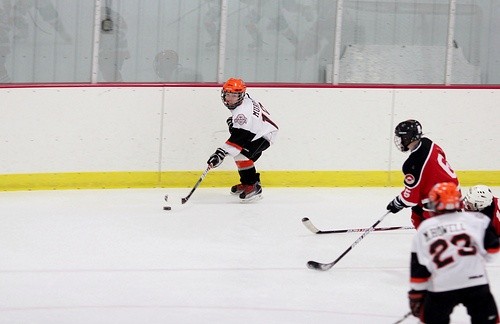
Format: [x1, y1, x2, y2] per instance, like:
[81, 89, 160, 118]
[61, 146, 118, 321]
[463, 185, 493, 212]
[429, 182, 461, 211]
[221, 78, 247, 110]
[394, 120, 423, 152]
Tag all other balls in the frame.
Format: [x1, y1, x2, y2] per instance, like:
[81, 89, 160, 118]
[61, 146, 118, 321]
[163, 207, 171, 210]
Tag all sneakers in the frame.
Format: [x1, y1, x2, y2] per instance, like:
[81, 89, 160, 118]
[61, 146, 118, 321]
[240, 173, 262, 203]
[230, 182, 247, 195]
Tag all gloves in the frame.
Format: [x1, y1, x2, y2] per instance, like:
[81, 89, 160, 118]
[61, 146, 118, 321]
[387, 196, 404, 213]
[409, 294, 423, 318]
[207, 148, 228, 168]
[227, 118, 233, 134]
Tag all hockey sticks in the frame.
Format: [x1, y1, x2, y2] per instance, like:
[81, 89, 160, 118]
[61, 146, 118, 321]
[302, 216, 417, 235]
[165, 163, 213, 205]
[307, 208, 393, 272]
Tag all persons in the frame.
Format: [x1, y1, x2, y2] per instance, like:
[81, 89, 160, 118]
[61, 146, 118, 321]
[207, 78, 279, 203]
[409, 182, 500, 324]
[387, 119, 464, 230]
